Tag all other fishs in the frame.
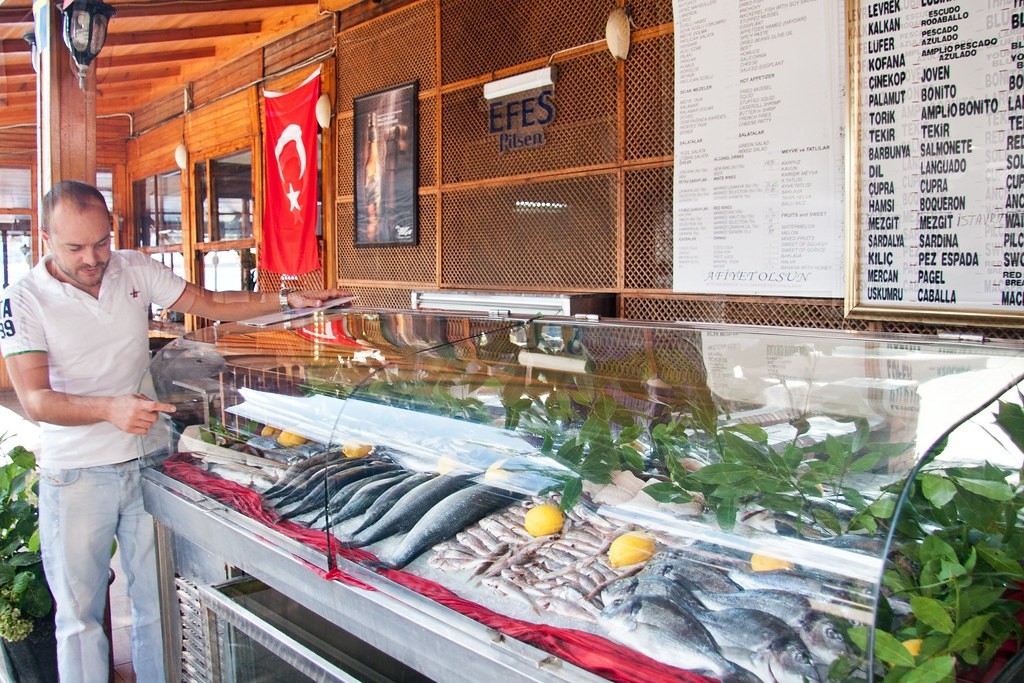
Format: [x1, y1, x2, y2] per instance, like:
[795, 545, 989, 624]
[261, 451, 859, 683]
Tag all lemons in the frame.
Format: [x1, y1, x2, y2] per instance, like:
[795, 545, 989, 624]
[526, 504, 563, 538]
[261, 424, 309, 447]
[608, 531, 655, 570]
[342, 438, 374, 459]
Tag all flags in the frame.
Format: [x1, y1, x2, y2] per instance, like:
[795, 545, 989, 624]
[261, 64, 321, 277]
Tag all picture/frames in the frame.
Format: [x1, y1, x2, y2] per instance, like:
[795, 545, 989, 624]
[352, 77, 420, 246]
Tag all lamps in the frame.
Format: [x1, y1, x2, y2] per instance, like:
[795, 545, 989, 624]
[57, 0, 117, 90]
[23, 32, 39, 72]
[483, 65, 557, 101]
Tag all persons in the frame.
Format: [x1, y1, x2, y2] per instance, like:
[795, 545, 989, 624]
[1, 181, 356, 683]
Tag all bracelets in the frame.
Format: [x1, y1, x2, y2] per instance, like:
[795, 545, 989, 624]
[280, 288, 297, 310]
